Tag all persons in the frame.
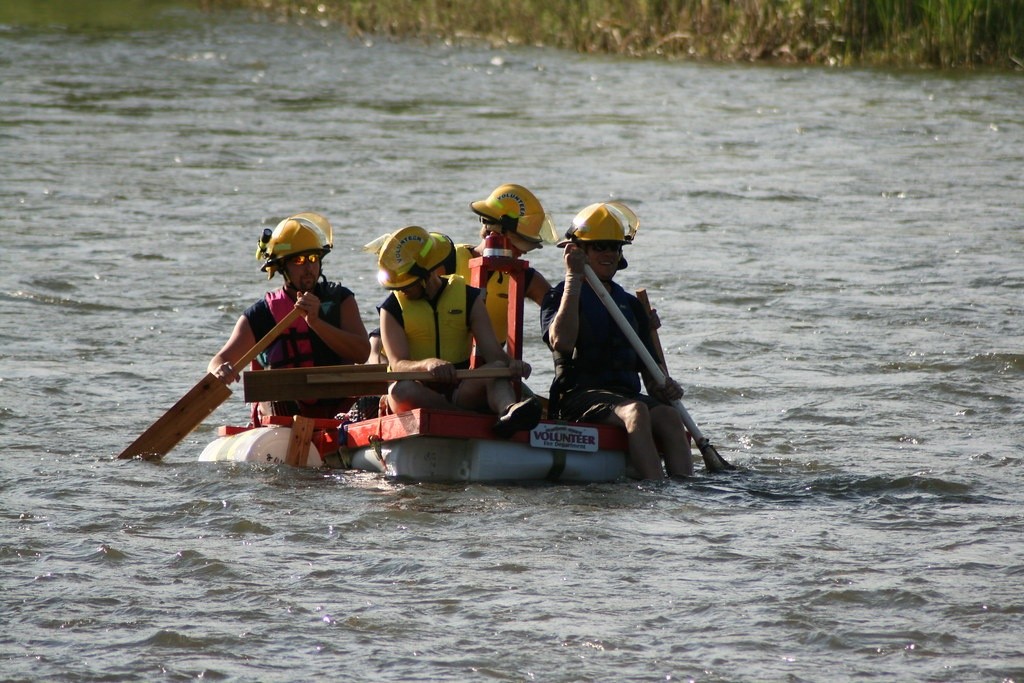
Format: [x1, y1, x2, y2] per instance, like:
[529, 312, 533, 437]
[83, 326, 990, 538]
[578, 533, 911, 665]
[376, 232, 545, 439]
[206, 183, 694, 481]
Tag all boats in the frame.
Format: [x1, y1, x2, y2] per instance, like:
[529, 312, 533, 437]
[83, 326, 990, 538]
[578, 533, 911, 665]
[198, 407, 691, 481]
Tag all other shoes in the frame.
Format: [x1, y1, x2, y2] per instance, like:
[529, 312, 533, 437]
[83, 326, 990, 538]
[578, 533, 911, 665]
[491, 397, 542, 440]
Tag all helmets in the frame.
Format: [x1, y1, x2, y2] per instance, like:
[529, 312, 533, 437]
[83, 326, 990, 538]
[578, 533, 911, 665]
[556, 203, 633, 247]
[377, 225, 453, 289]
[260, 215, 332, 271]
[469, 184, 544, 243]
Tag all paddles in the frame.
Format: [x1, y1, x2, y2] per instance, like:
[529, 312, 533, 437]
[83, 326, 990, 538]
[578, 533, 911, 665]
[634, 287, 670, 384]
[242, 363, 515, 403]
[580, 261, 732, 470]
[117, 305, 305, 460]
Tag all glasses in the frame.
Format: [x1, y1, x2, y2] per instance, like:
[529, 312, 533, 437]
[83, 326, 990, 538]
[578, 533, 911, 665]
[585, 240, 621, 252]
[286, 250, 321, 269]
